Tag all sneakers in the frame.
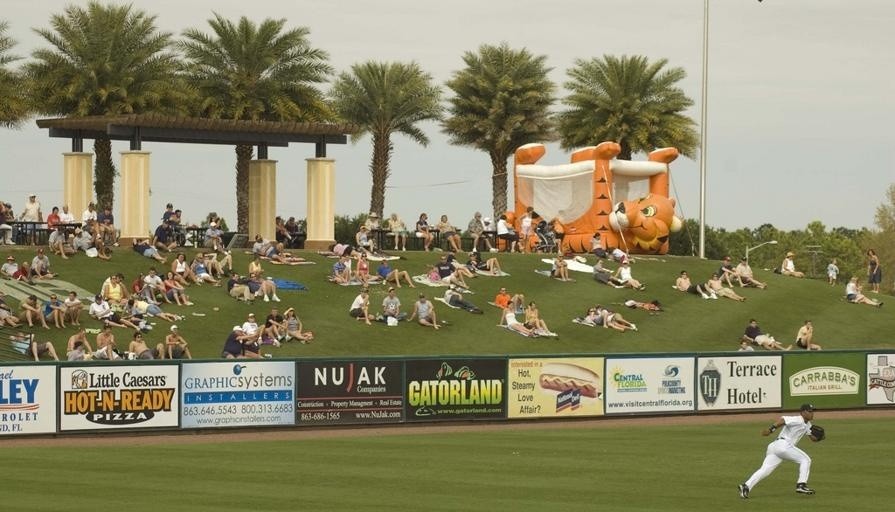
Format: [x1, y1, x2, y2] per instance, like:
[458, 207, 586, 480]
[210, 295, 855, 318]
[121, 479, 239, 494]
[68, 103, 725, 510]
[738, 484, 750, 499]
[271, 296, 280, 302]
[796, 484, 816, 495]
[263, 296, 270, 302]
[710, 293, 718, 300]
[702, 294, 709, 300]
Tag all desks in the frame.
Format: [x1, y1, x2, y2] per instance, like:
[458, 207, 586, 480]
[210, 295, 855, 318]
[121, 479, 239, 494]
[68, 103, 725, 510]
[178, 225, 208, 248]
[1, 218, 82, 246]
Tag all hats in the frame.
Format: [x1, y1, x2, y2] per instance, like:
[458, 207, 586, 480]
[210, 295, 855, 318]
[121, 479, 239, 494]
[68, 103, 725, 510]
[785, 252, 794, 258]
[360, 287, 369, 292]
[210, 222, 216, 227]
[370, 212, 378, 218]
[233, 326, 242, 331]
[7, 256, 15, 260]
[801, 403, 816, 411]
[95, 294, 103, 300]
[75, 229, 82, 234]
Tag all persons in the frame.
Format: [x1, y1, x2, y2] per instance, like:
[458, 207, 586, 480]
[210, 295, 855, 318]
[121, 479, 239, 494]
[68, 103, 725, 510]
[738, 317, 821, 350]
[736, 402, 826, 500]
[845, 277, 883, 307]
[676, 257, 767, 302]
[827, 258, 839, 287]
[319, 206, 661, 338]
[1, 194, 314, 359]
[867, 249, 881, 293]
[781, 252, 804, 278]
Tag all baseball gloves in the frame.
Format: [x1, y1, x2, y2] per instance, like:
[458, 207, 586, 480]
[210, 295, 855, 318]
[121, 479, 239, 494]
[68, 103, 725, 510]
[811, 424, 824, 440]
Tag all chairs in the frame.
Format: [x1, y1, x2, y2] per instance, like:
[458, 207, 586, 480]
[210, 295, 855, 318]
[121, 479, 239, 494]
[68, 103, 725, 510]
[362, 211, 499, 254]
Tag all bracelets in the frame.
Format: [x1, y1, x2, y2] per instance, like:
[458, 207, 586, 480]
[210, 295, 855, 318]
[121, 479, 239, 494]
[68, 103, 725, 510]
[769, 423, 777, 433]
